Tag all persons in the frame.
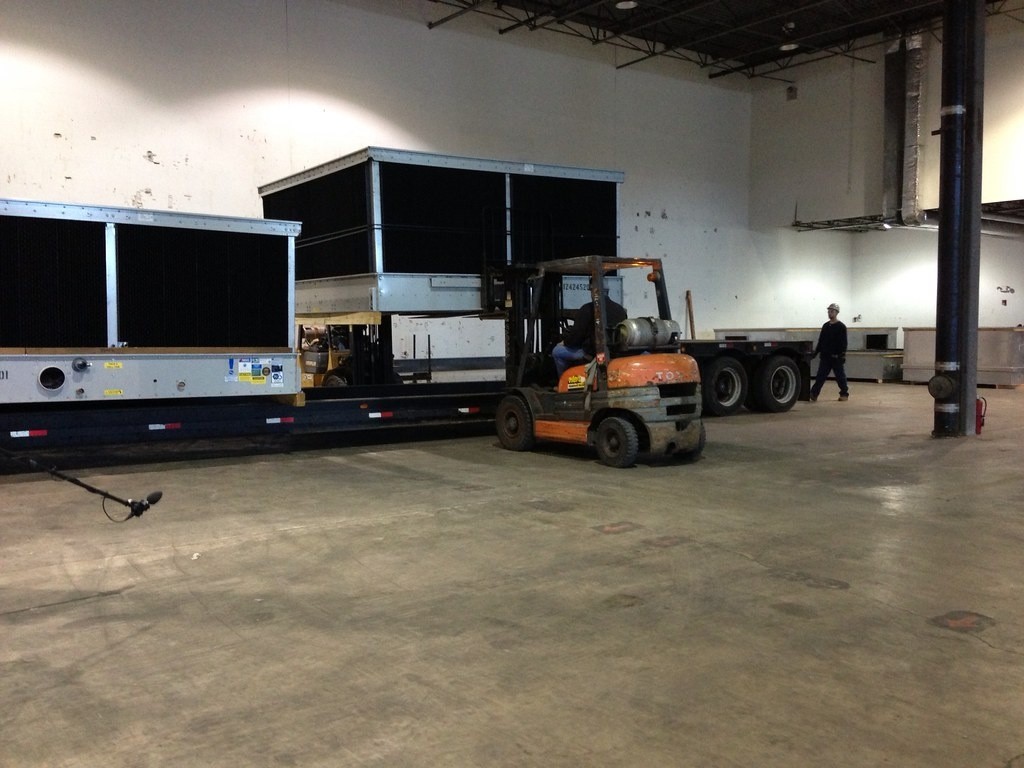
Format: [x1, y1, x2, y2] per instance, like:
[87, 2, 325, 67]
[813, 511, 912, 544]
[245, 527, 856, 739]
[551, 277, 628, 380]
[810, 303, 850, 401]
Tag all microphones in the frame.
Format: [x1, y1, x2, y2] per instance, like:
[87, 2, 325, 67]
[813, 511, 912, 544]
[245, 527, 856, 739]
[124, 491, 163, 521]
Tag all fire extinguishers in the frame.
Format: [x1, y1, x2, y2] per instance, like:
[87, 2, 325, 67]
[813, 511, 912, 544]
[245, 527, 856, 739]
[975, 393, 987, 435]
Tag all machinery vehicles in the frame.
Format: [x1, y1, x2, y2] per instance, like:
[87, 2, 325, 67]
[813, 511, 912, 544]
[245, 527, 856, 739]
[295, 311, 404, 387]
[463, 255, 706, 468]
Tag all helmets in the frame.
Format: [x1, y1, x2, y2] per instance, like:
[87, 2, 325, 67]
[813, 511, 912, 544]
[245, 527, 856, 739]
[827, 303, 840, 312]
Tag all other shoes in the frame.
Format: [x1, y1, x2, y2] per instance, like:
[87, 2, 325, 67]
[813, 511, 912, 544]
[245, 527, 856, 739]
[839, 395, 848, 401]
[809, 392, 816, 401]
